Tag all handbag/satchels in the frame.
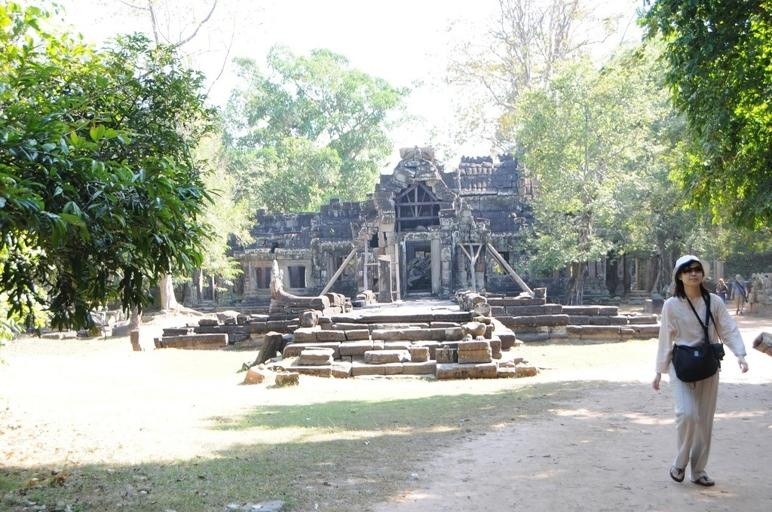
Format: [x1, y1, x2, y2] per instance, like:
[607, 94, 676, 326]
[672, 343, 717, 381]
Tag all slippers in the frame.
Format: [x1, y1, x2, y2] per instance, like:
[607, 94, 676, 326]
[670, 465, 686, 482]
[691, 475, 714, 486]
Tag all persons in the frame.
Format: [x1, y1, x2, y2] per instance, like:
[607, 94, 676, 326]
[715, 274, 772, 316]
[654, 256, 748, 487]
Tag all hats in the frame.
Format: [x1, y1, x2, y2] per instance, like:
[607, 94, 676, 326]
[672, 255, 704, 282]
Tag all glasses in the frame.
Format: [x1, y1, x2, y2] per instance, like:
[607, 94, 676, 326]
[683, 266, 702, 272]
